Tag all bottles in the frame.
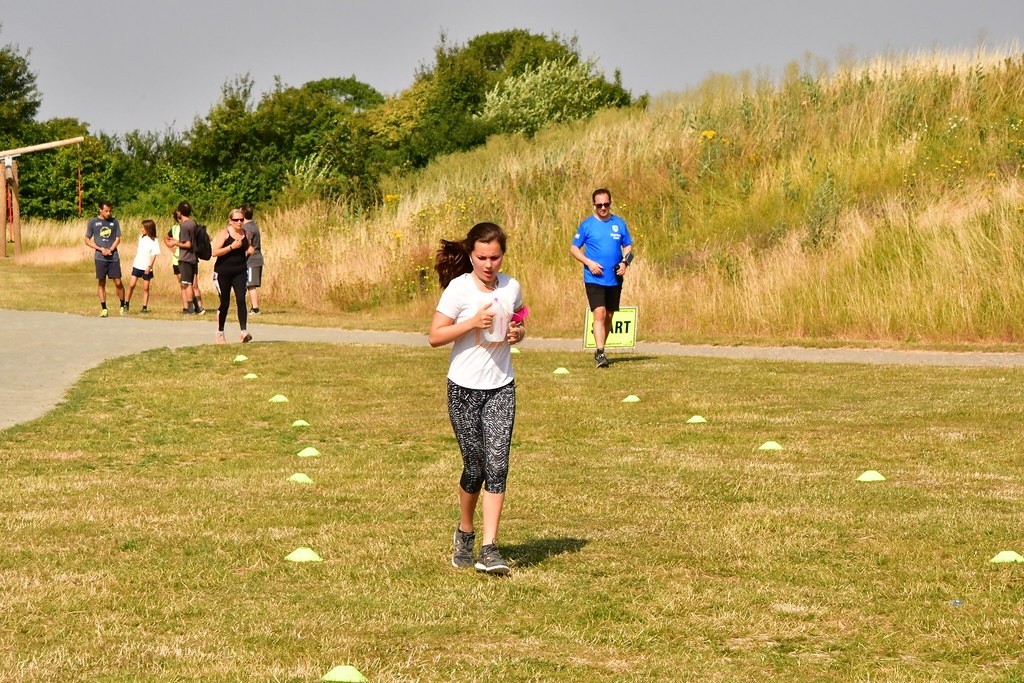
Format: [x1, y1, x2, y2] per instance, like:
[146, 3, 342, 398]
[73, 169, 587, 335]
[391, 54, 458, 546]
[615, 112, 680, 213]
[483, 298, 507, 341]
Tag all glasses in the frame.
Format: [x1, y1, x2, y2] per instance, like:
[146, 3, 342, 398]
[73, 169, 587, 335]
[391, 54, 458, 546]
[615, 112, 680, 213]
[595, 203, 611, 209]
[231, 219, 245, 223]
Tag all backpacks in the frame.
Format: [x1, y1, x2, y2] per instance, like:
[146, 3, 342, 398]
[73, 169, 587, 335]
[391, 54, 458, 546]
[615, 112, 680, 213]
[192, 219, 212, 260]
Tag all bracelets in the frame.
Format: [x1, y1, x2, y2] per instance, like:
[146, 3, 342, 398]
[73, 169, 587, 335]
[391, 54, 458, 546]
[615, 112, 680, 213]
[519, 326, 526, 339]
[148, 266, 152, 268]
[229, 245, 232, 250]
[108, 248, 112, 251]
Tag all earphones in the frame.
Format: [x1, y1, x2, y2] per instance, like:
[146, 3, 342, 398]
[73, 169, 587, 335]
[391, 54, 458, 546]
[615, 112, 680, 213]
[469, 257, 474, 267]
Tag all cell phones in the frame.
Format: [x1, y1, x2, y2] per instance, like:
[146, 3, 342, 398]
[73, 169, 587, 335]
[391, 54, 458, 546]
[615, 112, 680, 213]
[626, 252, 633, 265]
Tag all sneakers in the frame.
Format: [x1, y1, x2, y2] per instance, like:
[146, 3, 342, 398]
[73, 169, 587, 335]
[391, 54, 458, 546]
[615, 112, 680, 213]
[451, 523, 475, 569]
[475, 537, 510, 573]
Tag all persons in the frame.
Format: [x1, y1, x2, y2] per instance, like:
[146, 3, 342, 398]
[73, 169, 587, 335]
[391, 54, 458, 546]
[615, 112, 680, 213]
[84, 201, 126, 317]
[124, 219, 161, 312]
[209, 208, 253, 344]
[164, 200, 206, 316]
[569, 189, 635, 367]
[241, 206, 264, 315]
[425, 221, 527, 574]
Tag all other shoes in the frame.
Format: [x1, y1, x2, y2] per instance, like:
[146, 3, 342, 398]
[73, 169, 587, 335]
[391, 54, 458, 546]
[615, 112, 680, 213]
[119, 307, 124, 315]
[123, 304, 129, 312]
[239, 330, 253, 343]
[141, 309, 148, 313]
[100, 308, 108, 317]
[247, 306, 261, 315]
[594, 352, 609, 368]
[217, 330, 226, 344]
[178, 309, 189, 315]
[193, 308, 206, 316]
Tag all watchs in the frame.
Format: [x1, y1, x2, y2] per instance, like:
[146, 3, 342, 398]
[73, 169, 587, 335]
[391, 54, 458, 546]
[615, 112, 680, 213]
[622, 260, 628, 267]
